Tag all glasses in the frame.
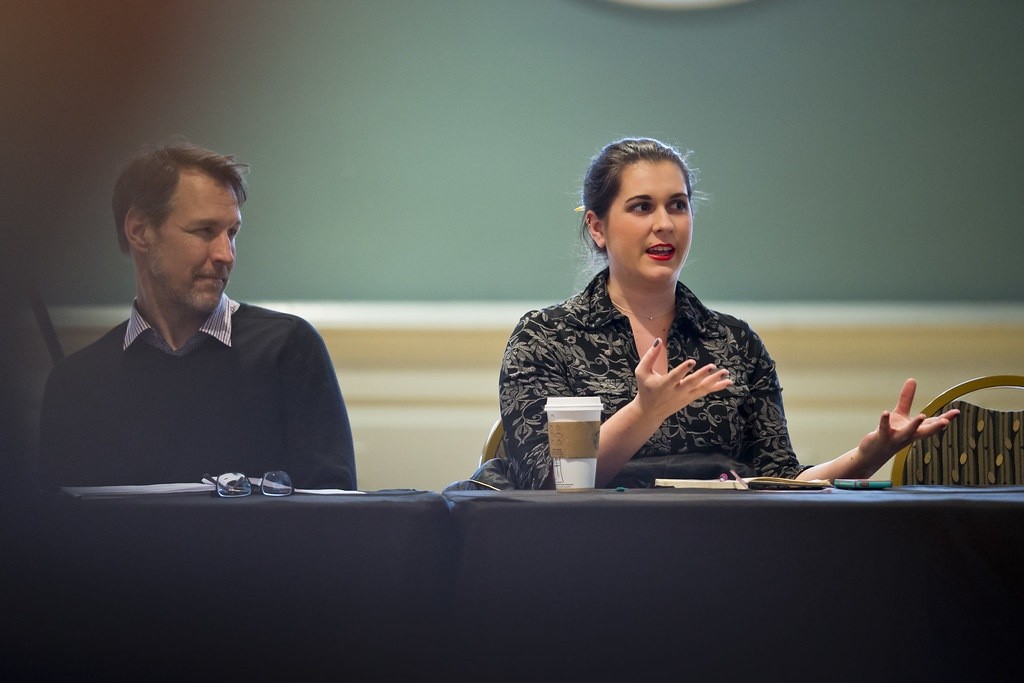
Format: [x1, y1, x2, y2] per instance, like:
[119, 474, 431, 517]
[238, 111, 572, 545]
[200, 471, 297, 498]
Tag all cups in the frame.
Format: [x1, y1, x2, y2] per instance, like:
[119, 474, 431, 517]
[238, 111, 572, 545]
[544, 397, 605, 492]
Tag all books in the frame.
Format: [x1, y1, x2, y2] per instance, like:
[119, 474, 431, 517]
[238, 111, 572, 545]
[63, 476, 367, 501]
[654, 475, 832, 493]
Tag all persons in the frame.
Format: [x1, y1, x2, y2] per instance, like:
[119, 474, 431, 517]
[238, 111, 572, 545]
[498, 136, 961, 495]
[31, 142, 356, 501]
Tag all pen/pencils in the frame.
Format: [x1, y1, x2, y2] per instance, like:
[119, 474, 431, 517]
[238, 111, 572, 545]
[719, 474, 728, 482]
[730, 470, 748, 488]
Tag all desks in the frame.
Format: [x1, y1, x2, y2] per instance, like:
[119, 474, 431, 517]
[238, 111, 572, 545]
[0, 487, 1024, 683]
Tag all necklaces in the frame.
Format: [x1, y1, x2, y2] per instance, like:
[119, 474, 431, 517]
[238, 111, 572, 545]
[612, 301, 675, 319]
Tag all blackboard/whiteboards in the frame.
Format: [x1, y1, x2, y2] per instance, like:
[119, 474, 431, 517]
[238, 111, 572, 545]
[0, 0, 1024, 331]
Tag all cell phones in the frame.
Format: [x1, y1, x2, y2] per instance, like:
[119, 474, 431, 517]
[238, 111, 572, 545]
[835, 479, 893, 490]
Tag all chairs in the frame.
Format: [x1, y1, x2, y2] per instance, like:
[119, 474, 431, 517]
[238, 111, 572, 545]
[891, 374, 1024, 485]
